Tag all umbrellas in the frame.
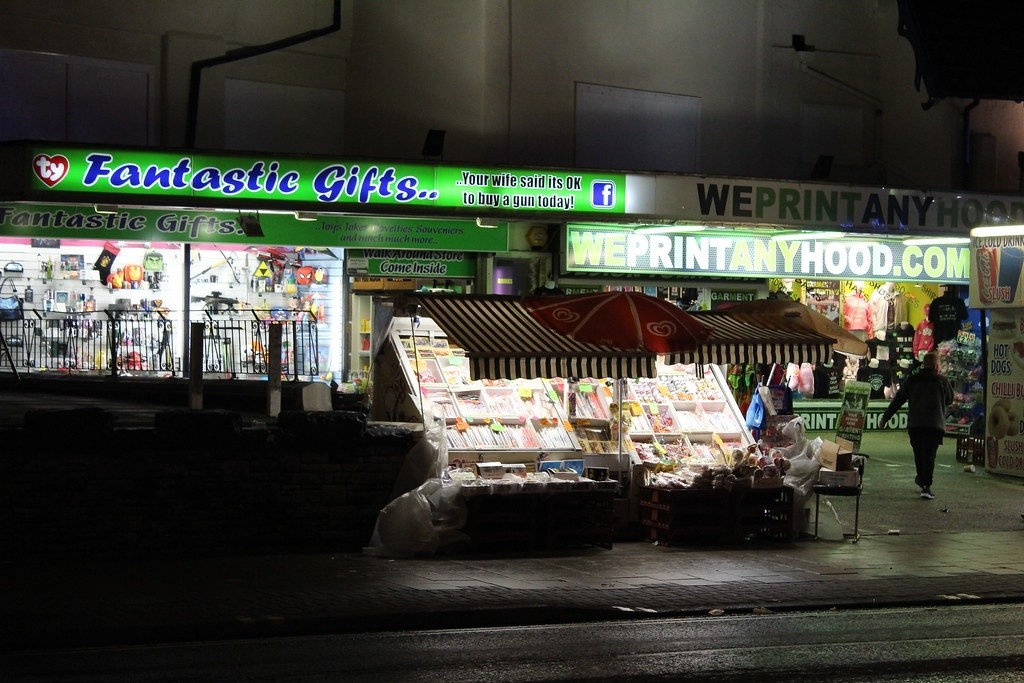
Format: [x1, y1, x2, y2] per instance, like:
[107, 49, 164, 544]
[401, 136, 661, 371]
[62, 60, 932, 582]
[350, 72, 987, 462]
[533, 290, 714, 461]
[717, 295, 868, 387]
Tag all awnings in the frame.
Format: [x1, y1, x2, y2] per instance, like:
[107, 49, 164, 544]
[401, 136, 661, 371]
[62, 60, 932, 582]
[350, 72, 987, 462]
[400, 292, 656, 378]
[664, 308, 830, 371]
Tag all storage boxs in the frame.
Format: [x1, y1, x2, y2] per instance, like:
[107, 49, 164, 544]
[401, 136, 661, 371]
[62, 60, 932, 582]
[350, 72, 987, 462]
[818, 436, 854, 471]
[79, 269, 101, 280]
[819, 467, 861, 486]
[392, 329, 796, 550]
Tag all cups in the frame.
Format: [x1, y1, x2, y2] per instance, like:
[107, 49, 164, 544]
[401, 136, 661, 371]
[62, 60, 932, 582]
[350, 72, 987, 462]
[998, 245, 1024, 303]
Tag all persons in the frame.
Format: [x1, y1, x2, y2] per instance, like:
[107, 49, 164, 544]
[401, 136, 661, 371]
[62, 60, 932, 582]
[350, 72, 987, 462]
[880, 354, 955, 500]
[843, 281, 873, 342]
[857, 314, 933, 399]
[815, 360, 843, 399]
[871, 281, 906, 335]
[928, 290, 968, 349]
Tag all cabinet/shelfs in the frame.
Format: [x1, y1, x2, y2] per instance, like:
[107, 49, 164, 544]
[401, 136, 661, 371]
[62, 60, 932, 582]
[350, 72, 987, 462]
[358, 295, 374, 382]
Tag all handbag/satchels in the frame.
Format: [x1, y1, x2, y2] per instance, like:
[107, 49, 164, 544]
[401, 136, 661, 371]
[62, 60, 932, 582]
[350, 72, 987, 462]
[745, 385, 765, 430]
[0, 277, 24, 320]
[142, 250, 163, 270]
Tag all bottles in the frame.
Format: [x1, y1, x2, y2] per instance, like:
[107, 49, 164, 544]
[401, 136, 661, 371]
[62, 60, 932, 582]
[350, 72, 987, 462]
[25, 285, 33, 302]
[47, 259, 52, 278]
[145, 298, 149, 311]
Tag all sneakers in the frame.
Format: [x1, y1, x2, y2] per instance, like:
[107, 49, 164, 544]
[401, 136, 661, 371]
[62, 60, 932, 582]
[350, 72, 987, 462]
[915, 476, 926, 493]
[921, 488, 935, 498]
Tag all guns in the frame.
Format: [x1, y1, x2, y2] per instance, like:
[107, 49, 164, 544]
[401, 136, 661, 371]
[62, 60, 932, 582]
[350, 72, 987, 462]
[191, 291, 239, 315]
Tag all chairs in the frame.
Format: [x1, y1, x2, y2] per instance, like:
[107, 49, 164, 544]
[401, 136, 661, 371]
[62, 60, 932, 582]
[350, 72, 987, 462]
[810, 452, 870, 545]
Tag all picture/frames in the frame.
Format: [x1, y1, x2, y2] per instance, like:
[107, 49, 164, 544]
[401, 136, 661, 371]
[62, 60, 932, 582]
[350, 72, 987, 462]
[54, 290, 70, 306]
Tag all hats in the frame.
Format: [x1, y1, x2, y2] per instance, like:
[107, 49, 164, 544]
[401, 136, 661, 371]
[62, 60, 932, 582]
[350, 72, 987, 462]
[924, 303, 930, 315]
